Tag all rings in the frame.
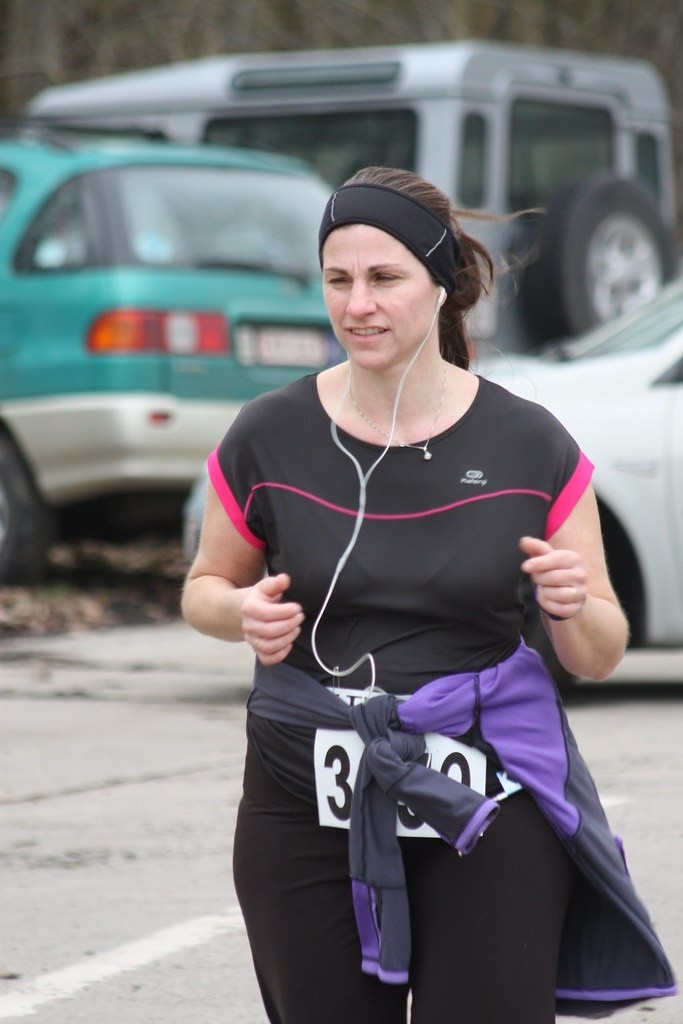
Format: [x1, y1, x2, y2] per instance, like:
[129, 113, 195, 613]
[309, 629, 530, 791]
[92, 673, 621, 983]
[253, 639, 261, 648]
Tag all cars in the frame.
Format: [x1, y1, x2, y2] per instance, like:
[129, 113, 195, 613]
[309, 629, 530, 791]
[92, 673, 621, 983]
[0, 123, 358, 584]
[469, 279, 683, 690]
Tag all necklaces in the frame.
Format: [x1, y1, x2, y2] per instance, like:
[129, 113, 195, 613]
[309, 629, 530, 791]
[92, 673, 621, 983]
[347, 358, 448, 460]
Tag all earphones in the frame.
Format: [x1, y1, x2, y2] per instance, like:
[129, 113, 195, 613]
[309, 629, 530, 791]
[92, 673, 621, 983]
[435, 285, 446, 314]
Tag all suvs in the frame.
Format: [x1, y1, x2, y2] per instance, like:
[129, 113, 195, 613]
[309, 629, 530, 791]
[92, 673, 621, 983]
[14, 38, 678, 365]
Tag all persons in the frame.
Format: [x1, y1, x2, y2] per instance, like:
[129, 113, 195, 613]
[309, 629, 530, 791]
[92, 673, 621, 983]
[179, 167, 630, 1024]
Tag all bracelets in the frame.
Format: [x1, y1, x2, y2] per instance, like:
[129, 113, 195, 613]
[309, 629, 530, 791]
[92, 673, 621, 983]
[536, 584, 585, 622]
